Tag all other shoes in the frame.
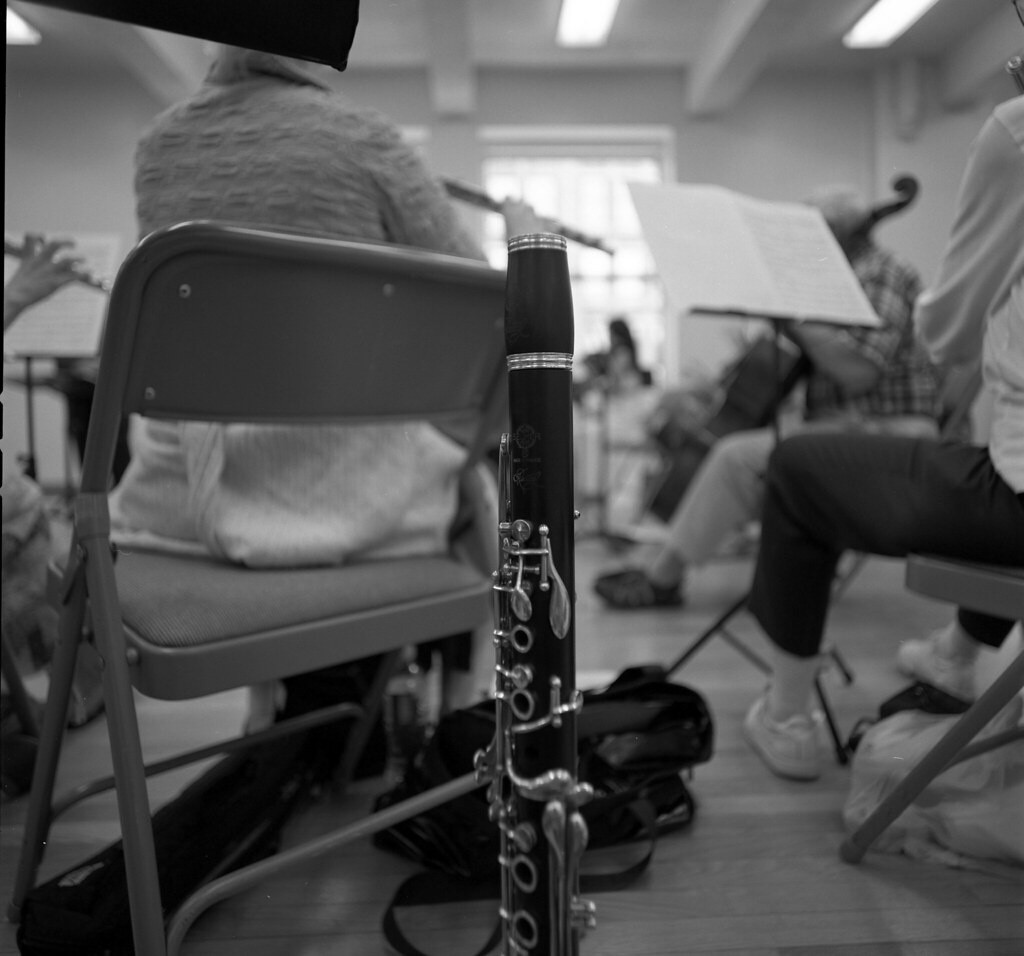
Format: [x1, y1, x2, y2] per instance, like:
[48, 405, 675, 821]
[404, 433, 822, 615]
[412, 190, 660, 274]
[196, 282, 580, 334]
[594, 570, 685, 607]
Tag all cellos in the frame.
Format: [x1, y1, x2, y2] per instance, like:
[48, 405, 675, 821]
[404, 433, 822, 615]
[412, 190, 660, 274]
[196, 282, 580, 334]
[640, 171, 918, 534]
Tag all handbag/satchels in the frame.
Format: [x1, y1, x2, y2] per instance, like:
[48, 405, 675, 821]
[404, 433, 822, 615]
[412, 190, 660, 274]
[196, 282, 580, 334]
[845, 621, 1024, 864]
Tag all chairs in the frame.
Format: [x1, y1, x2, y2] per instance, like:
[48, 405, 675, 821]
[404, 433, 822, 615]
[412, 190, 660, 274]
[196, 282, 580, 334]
[5, 218, 512, 956]
[837, 547, 1023, 868]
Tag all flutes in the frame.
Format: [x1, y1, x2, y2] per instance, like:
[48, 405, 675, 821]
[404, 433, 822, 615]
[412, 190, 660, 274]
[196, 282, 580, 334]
[476, 227, 601, 955]
[435, 176, 621, 259]
[2, 236, 113, 299]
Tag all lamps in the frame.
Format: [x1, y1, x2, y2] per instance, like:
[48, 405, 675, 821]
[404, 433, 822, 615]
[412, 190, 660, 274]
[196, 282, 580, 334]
[554, 0, 622, 47]
[5, 8, 42, 48]
[839, 1, 939, 53]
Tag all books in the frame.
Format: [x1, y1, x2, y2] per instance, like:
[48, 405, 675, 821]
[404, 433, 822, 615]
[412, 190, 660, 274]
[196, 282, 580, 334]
[627, 182, 883, 324]
[6, 276, 108, 354]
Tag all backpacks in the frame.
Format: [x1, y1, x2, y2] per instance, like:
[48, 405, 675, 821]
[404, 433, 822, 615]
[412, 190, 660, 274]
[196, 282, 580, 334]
[373, 666, 714, 956]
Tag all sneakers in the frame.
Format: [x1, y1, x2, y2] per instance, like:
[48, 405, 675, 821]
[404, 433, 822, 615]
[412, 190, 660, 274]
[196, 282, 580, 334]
[744, 697, 821, 780]
[897, 629, 976, 705]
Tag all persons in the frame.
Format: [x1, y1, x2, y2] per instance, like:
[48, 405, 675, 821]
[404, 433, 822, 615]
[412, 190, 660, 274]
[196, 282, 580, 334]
[745, 0, 1024, 776]
[6, 228, 110, 731]
[125, 33, 498, 763]
[587, 186, 940, 613]
[593, 318, 644, 555]
